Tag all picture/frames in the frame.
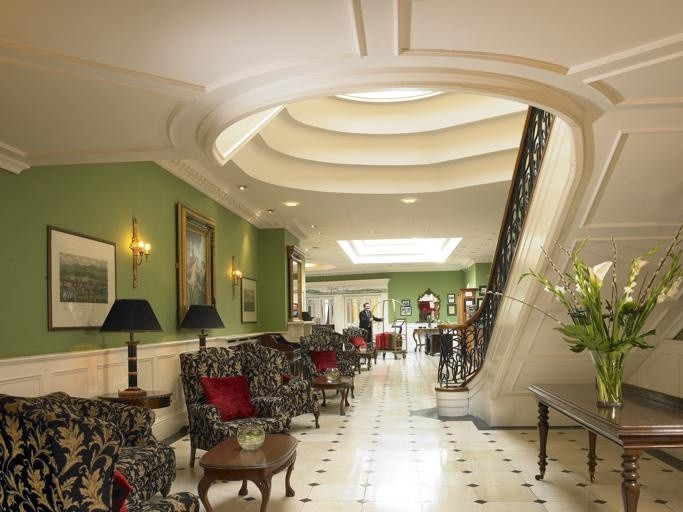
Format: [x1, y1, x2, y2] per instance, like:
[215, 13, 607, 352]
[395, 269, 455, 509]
[174, 199, 218, 333]
[399, 300, 412, 317]
[239, 274, 258, 324]
[445, 285, 489, 323]
[43, 223, 119, 334]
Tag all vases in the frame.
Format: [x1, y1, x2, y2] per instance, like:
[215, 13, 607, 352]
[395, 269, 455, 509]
[586, 346, 627, 410]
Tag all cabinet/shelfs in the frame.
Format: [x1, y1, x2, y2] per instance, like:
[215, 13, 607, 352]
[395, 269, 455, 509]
[423, 332, 453, 358]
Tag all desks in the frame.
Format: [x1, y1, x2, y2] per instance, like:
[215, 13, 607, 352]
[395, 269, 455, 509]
[99, 391, 172, 415]
[522, 379, 681, 512]
[413, 328, 440, 353]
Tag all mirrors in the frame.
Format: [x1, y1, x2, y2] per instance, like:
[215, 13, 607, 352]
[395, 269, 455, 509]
[416, 287, 441, 323]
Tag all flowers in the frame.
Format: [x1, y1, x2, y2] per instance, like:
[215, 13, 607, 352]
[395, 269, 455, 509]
[477, 225, 682, 404]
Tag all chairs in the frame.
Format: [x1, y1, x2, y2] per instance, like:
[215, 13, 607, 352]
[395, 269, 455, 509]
[172, 322, 383, 469]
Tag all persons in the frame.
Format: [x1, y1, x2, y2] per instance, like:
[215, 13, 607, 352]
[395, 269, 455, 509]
[359, 303, 383, 348]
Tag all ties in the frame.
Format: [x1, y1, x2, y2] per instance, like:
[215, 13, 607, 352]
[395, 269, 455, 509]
[368, 311, 370, 327]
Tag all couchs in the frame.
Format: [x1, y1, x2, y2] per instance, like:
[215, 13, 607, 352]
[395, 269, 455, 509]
[0, 392, 202, 512]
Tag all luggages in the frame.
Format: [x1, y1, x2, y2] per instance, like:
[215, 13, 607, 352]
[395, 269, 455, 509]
[376, 332, 402, 351]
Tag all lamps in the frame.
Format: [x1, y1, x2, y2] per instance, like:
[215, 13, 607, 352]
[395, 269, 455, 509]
[230, 255, 242, 300]
[178, 304, 225, 355]
[128, 216, 151, 290]
[97, 298, 165, 396]
